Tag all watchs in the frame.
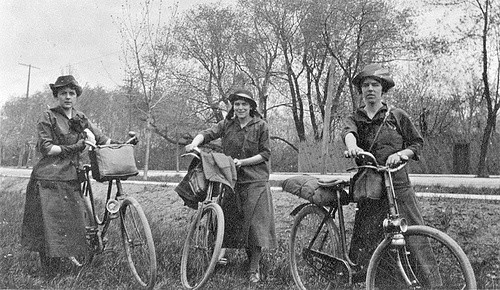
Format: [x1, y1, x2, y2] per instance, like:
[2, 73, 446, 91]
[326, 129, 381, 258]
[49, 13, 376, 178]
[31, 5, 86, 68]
[397, 151, 402, 156]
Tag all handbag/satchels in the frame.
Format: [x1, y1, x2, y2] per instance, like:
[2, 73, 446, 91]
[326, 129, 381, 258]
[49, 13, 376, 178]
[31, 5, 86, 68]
[349, 108, 391, 201]
[89, 142, 138, 182]
[189, 163, 219, 197]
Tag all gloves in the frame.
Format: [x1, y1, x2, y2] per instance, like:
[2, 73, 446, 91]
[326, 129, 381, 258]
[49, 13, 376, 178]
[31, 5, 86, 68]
[61, 139, 86, 156]
[111, 138, 139, 145]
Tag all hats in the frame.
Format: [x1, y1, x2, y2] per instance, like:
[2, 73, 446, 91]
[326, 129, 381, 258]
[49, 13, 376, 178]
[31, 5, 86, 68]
[228, 90, 257, 110]
[352, 64, 394, 90]
[49, 75, 82, 98]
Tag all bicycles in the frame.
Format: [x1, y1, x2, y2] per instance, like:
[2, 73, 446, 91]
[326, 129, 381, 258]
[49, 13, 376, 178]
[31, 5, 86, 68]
[282, 151, 477, 290]
[180, 147, 227, 290]
[68, 131, 157, 290]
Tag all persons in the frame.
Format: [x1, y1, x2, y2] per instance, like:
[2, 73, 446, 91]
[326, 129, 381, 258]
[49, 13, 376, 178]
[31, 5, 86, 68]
[339, 63, 445, 290]
[20, 74, 140, 274]
[183, 89, 278, 284]
